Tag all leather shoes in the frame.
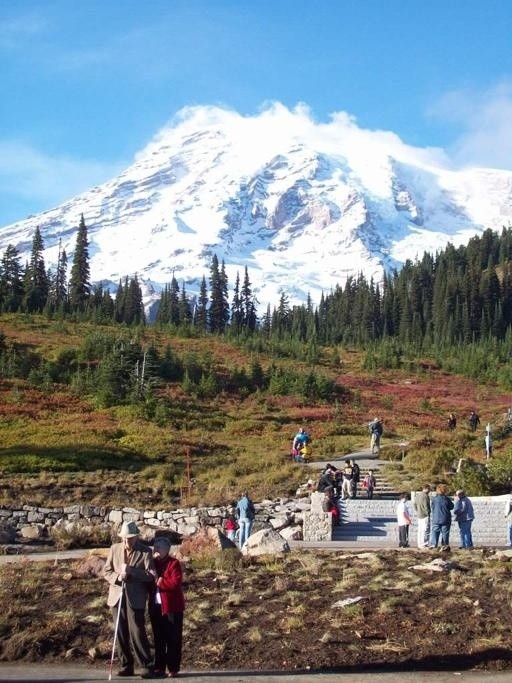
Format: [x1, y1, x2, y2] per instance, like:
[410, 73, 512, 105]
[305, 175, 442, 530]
[140, 666, 152, 679]
[115, 667, 134, 676]
[168, 672, 177, 677]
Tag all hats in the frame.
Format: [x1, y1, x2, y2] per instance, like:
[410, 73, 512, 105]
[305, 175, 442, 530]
[117, 520, 140, 538]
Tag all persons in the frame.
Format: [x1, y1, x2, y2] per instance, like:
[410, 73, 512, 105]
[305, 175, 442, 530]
[317, 458, 379, 526]
[105, 522, 164, 677]
[448, 414, 456, 428]
[145, 528, 185, 680]
[505, 492, 512, 545]
[293, 428, 313, 463]
[468, 411, 480, 431]
[224, 505, 238, 544]
[235, 492, 254, 549]
[369, 418, 383, 454]
[396, 483, 474, 551]
